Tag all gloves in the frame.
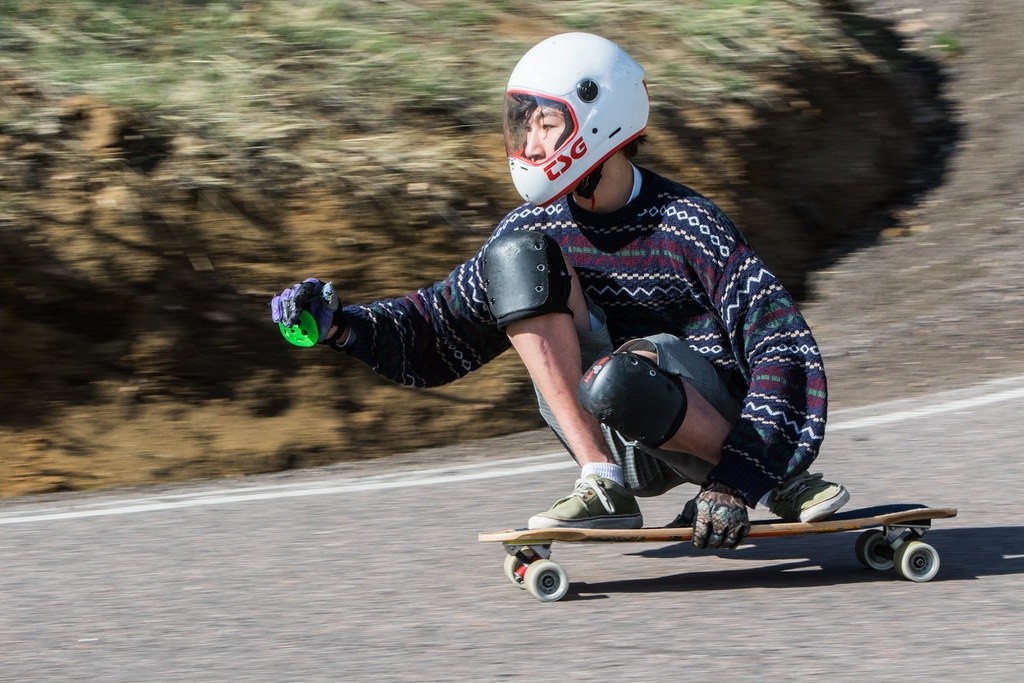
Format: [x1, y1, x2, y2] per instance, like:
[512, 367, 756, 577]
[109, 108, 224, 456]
[271, 276, 345, 346]
[663, 479, 753, 550]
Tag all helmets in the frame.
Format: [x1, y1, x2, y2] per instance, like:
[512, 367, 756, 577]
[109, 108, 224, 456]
[504, 32, 651, 206]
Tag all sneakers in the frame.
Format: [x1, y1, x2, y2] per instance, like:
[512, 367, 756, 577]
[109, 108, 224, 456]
[767, 471, 850, 523]
[527, 474, 644, 531]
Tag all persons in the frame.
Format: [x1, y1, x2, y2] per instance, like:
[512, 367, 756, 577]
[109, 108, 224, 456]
[271, 32, 851, 550]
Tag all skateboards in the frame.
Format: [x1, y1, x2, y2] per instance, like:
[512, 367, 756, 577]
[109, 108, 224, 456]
[476, 502, 959, 603]
